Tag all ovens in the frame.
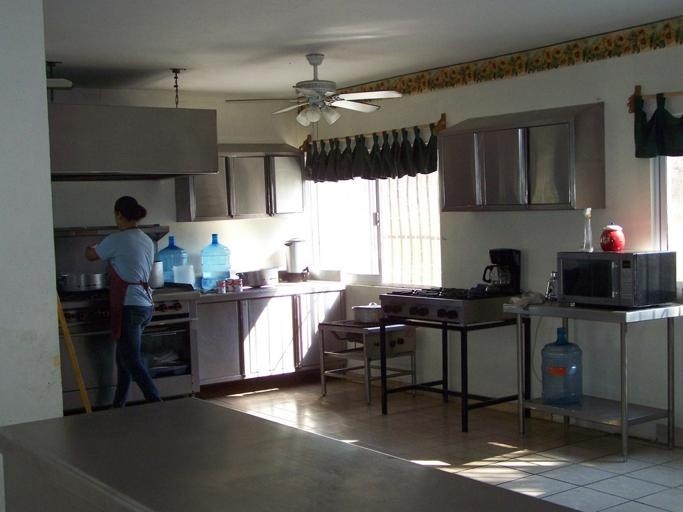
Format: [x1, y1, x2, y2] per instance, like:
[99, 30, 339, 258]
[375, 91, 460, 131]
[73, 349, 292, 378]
[63, 312, 200, 415]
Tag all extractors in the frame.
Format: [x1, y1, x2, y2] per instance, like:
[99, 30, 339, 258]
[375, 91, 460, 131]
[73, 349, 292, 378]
[46, 104, 222, 183]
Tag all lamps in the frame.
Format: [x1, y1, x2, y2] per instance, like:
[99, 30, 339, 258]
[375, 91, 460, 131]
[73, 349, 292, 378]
[304, 104, 322, 126]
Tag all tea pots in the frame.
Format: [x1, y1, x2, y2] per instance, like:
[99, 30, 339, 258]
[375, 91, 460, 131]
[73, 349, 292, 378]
[543, 272, 558, 302]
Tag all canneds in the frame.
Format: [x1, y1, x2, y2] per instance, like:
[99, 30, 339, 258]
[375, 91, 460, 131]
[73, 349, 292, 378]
[217, 279, 243, 294]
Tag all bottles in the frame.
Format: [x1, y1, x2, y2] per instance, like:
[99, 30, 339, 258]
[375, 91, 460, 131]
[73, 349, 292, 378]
[581, 206, 593, 252]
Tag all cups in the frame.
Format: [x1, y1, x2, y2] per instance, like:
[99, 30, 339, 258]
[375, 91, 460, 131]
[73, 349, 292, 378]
[215, 278, 243, 294]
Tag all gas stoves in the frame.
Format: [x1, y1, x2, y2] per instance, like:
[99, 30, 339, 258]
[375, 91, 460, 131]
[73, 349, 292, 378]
[61, 287, 200, 335]
[379, 285, 523, 324]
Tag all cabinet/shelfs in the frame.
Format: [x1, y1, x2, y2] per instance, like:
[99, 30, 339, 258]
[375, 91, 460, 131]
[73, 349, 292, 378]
[436, 115, 481, 215]
[295, 281, 348, 372]
[472, 111, 528, 214]
[268, 142, 307, 218]
[242, 283, 300, 381]
[174, 142, 234, 224]
[316, 318, 417, 405]
[196, 291, 246, 386]
[57, 294, 132, 412]
[226, 141, 273, 220]
[526, 102, 607, 213]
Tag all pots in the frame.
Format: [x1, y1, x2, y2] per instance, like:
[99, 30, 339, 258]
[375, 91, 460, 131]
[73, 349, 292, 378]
[351, 301, 382, 325]
[61, 270, 108, 292]
[236, 266, 280, 286]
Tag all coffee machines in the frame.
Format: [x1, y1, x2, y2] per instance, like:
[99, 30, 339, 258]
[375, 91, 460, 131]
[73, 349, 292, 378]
[482, 247, 522, 295]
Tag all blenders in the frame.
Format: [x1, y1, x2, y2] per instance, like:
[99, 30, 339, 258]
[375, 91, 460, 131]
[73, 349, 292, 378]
[285, 233, 311, 282]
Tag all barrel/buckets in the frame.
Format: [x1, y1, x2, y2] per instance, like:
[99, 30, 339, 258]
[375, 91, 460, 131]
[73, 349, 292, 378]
[156, 234, 188, 282]
[541, 327, 583, 405]
[200, 233, 230, 292]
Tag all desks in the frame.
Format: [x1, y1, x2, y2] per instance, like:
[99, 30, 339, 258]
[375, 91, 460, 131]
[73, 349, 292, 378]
[0, 395, 578, 512]
[495, 298, 682, 466]
[372, 315, 533, 433]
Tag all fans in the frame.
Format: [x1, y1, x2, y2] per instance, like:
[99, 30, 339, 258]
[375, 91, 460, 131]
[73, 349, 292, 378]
[223, 52, 405, 127]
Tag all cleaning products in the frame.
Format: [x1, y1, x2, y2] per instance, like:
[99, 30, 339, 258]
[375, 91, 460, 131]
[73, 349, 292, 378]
[584, 208, 594, 253]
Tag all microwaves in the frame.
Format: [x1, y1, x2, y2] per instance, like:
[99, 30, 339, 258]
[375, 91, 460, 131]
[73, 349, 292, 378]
[557, 250, 678, 308]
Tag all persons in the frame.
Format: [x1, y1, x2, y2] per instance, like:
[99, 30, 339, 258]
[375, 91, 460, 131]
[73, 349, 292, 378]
[84, 196, 163, 408]
